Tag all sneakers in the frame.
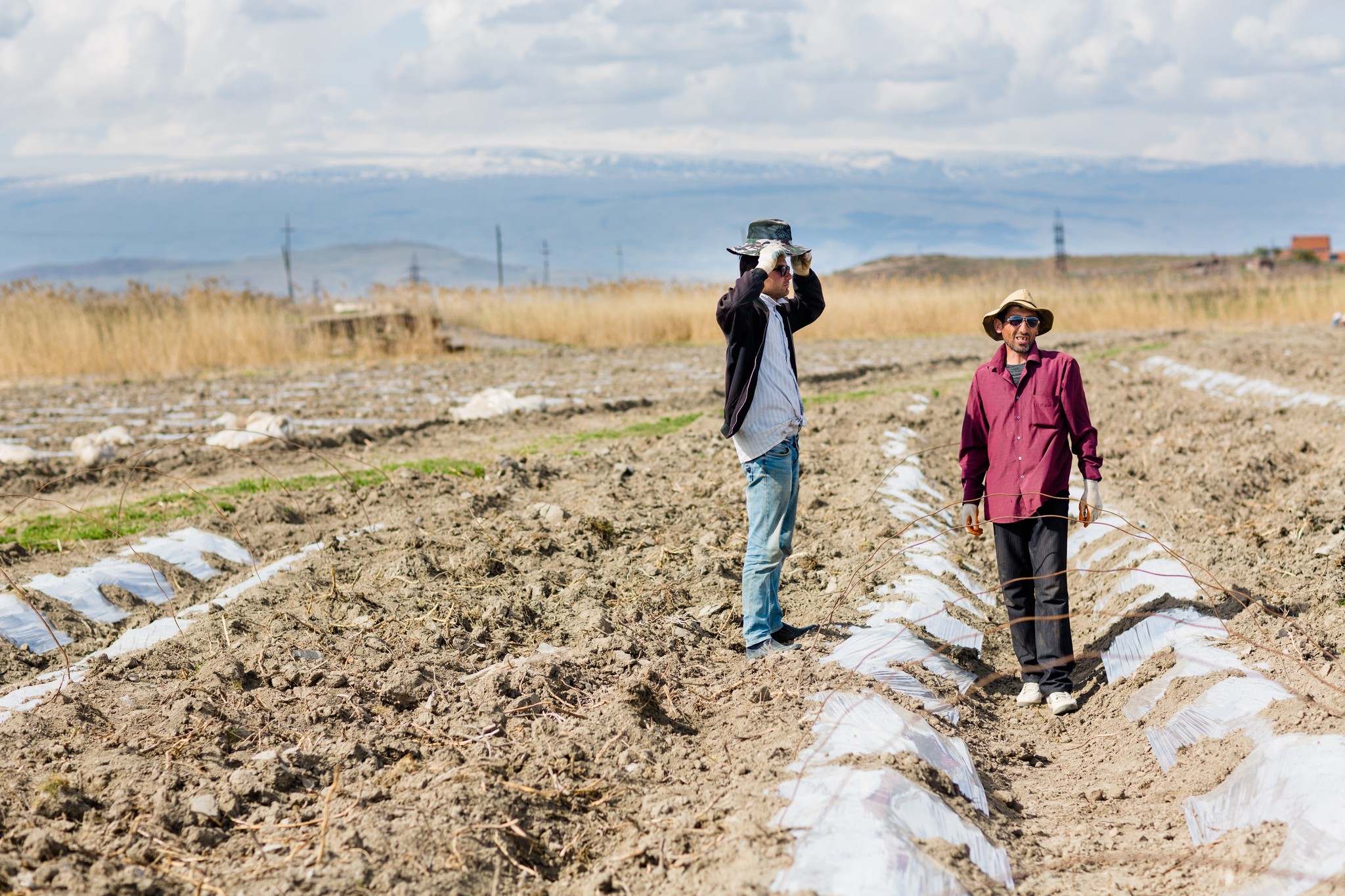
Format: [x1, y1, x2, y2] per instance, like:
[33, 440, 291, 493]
[1046, 692, 1078, 715]
[1016, 682, 1044, 707]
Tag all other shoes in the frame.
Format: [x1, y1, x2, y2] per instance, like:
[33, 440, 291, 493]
[745, 638, 802, 661]
[772, 622, 817, 644]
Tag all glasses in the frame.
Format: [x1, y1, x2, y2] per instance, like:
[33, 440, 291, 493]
[1001, 316, 1040, 328]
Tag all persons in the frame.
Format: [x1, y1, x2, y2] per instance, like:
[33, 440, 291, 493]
[958, 289, 1104, 714]
[715, 218, 827, 660]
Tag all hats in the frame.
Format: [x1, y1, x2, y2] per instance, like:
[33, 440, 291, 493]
[726, 218, 811, 256]
[982, 289, 1054, 341]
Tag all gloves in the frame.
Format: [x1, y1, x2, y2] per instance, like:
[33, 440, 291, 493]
[1079, 479, 1103, 528]
[755, 244, 787, 274]
[790, 245, 812, 276]
[961, 503, 983, 536]
